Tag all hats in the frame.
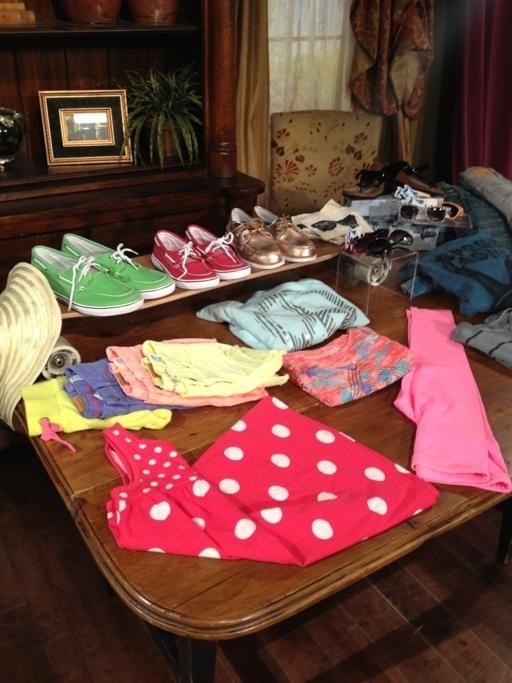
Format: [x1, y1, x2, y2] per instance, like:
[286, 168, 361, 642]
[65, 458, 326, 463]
[1, 260, 63, 432]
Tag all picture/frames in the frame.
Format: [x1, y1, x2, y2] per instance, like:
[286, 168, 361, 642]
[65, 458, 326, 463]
[33, 88, 136, 169]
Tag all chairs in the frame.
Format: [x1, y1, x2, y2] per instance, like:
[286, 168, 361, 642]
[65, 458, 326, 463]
[266, 108, 388, 218]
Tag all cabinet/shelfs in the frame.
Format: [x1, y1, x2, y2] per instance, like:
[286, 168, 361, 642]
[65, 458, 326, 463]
[1, 0, 264, 271]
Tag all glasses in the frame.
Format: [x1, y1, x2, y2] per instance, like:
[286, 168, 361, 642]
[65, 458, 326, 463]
[437, 201, 465, 221]
[347, 228, 414, 257]
[311, 213, 358, 231]
[399, 205, 446, 223]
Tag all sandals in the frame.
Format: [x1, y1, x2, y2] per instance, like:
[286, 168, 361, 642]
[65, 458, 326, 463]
[395, 163, 443, 196]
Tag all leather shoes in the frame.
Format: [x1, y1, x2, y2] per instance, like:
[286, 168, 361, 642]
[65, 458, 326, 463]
[343, 175, 398, 200]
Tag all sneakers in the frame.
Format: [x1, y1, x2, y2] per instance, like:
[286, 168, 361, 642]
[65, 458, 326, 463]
[183, 223, 251, 280]
[60, 233, 176, 301]
[251, 205, 317, 264]
[225, 207, 285, 269]
[150, 229, 220, 290]
[31, 245, 145, 317]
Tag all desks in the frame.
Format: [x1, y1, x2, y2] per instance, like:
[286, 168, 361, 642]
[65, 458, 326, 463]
[3, 167, 512, 683]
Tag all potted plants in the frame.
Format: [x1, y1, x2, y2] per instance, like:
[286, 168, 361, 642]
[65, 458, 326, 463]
[110, 62, 206, 165]
